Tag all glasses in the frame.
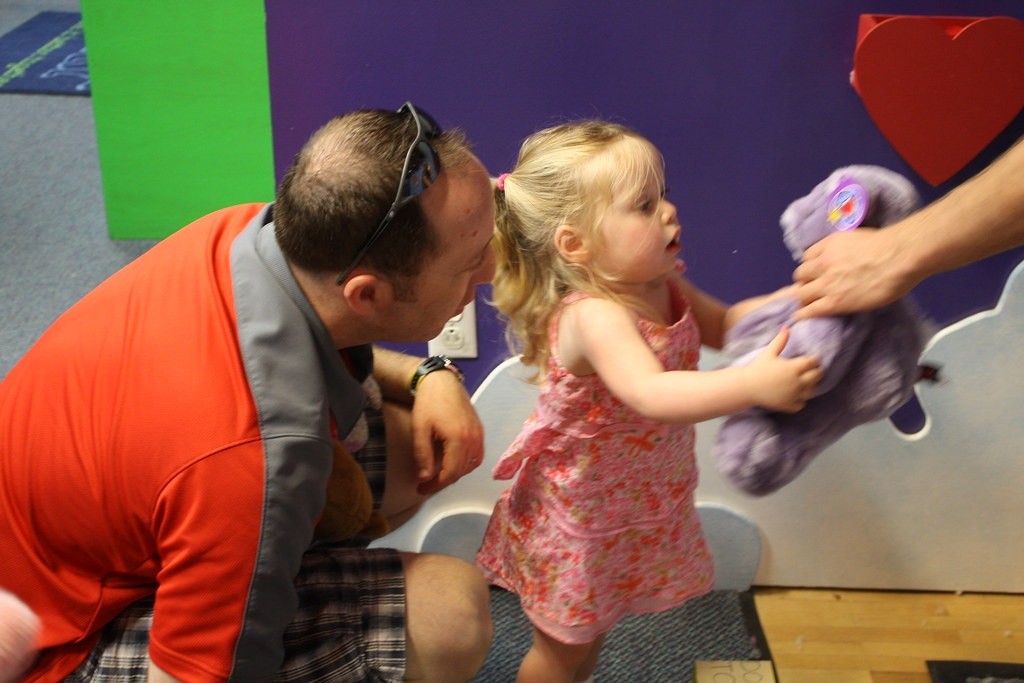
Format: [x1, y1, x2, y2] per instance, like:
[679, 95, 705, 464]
[336, 100, 443, 290]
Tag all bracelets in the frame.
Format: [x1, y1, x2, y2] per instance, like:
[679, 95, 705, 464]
[409, 354, 464, 397]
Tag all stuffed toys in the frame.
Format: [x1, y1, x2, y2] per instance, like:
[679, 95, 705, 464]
[718, 166, 942, 499]
[316, 376, 382, 543]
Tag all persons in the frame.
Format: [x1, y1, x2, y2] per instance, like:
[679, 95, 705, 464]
[0, 110, 495, 683]
[475, 121, 823, 683]
[787, 138, 1024, 320]
